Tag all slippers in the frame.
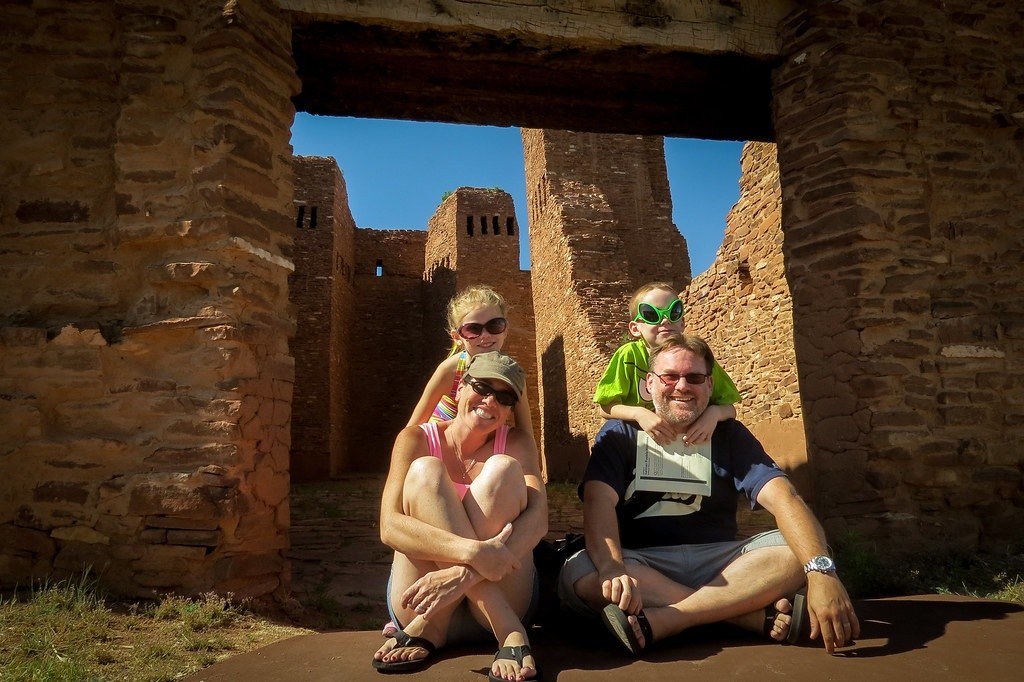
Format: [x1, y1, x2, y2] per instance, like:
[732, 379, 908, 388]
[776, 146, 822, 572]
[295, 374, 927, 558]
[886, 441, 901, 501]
[372, 630, 449, 672]
[761, 593, 806, 644]
[602, 603, 653, 654]
[489, 645, 535, 682]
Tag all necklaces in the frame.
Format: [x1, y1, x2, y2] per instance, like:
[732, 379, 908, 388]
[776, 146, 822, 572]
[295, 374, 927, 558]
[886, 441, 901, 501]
[448, 420, 481, 479]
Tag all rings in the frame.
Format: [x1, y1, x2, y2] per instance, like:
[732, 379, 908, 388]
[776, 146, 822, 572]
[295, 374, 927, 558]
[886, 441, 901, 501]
[418, 604, 426, 611]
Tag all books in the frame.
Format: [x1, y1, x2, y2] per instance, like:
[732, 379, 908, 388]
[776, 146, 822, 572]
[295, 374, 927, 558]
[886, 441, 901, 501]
[634, 429, 712, 496]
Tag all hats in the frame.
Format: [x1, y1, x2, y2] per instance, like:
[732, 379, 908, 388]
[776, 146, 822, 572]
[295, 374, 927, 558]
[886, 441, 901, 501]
[462, 350, 525, 402]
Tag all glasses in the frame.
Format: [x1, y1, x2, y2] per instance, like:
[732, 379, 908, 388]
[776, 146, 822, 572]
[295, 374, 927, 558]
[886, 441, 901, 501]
[455, 317, 507, 339]
[634, 299, 684, 325]
[463, 378, 519, 411]
[652, 370, 711, 386]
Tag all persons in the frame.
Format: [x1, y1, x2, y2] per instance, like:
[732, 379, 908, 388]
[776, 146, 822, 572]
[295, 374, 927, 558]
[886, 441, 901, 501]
[592, 281, 742, 446]
[557, 333, 860, 656]
[381, 285, 536, 637]
[372, 351, 550, 682]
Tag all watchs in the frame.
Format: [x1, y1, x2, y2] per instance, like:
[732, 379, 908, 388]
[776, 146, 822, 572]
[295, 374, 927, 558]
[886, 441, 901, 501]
[803, 553, 837, 575]
